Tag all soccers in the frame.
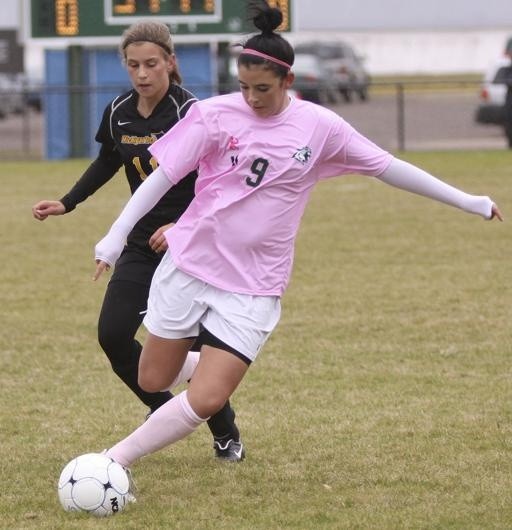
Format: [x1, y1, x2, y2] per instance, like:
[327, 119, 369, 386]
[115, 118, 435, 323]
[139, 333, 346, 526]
[58, 453, 129, 516]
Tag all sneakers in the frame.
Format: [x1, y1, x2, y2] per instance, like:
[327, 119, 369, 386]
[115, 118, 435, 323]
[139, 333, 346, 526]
[212, 436, 243, 463]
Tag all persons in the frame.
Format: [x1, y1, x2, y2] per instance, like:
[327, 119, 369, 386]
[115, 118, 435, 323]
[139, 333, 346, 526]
[93, 8, 504, 473]
[32, 23, 243, 465]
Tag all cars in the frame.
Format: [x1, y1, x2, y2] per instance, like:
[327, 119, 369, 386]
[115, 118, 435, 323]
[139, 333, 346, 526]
[480, 34, 511, 107]
[229, 39, 369, 104]
